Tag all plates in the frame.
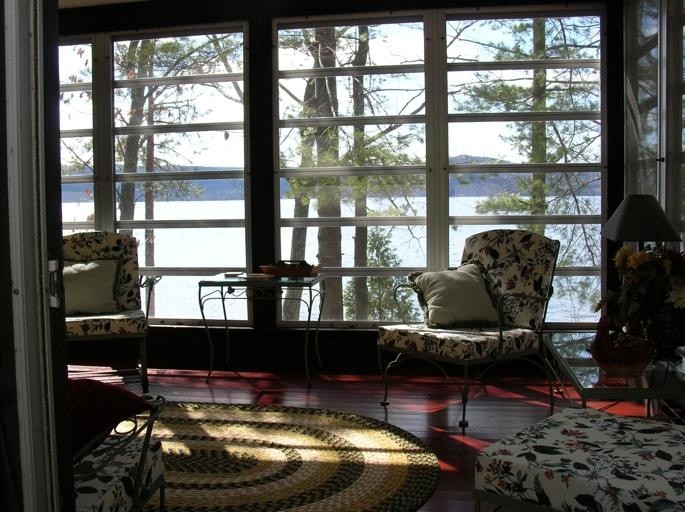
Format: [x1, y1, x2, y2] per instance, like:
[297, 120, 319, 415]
[239, 274, 276, 278]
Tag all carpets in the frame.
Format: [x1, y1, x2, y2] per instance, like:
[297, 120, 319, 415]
[108, 401, 440, 511]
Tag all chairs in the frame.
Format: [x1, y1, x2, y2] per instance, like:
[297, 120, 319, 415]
[473, 406, 685, 512]
[73, 395, 165, 512]
[63, 232, 148, 393]
[375, 229, 560, 427]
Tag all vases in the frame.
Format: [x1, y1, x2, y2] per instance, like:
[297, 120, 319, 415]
[643, 315, 685, 361]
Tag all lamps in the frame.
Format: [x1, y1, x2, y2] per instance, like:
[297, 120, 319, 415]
[600, 194, 683, 252]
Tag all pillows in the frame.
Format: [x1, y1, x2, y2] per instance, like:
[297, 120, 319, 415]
[69, 378, 151, 466]
[63, 259, 119, 316]
[414, 263, 499, 328]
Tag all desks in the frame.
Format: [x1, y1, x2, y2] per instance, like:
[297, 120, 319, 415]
[541, 332, 685, 421]
[198, 271, 327, 389]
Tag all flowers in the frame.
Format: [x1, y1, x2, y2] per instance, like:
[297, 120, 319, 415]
[596, 245, 685, 345]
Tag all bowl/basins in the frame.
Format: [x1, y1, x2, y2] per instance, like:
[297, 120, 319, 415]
[259, 265, 278, 273]
